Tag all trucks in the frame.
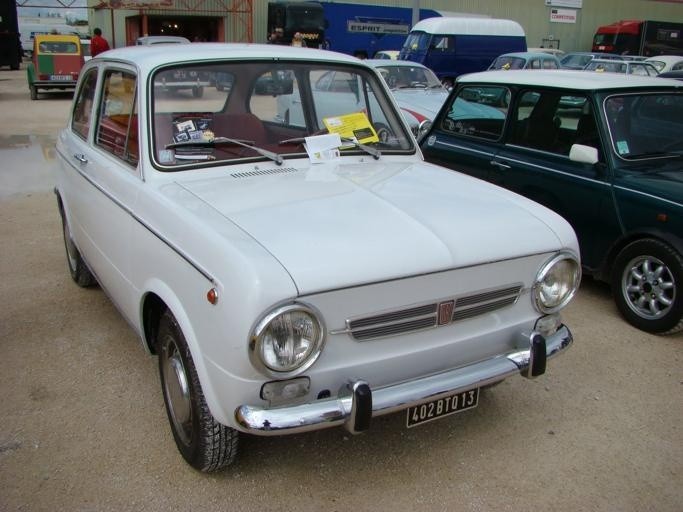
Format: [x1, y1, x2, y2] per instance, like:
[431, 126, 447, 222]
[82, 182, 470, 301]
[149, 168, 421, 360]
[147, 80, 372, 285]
[591, 18, 682, 56]
[267, 0, 330, 52]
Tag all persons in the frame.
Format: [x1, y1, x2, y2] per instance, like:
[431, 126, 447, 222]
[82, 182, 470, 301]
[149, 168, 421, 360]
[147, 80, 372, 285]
[89, 27, 111, 58]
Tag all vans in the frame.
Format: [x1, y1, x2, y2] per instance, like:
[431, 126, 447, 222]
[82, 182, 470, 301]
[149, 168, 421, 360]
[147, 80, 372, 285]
[17, 24, 80, 53]
[395, 17, 527, 91]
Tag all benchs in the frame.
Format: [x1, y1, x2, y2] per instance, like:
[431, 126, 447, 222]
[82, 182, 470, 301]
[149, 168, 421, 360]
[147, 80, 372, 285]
[100, 113, 267, 158]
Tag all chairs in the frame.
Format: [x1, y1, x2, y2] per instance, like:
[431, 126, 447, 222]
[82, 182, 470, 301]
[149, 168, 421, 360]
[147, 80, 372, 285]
[519, 115, 558, 152]
[575, 114, 617, 151]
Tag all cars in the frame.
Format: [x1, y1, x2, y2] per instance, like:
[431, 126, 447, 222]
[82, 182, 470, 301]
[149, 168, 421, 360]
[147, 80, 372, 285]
[79, 38, 91, 63]
[454, 48, 683, 115]
[415, 68, 683, 334]
[372, 50, 400, 60]
[26, 34, 85, 101]
[213, 65, 294, 94]
[274, 59, 507, 147]
[120, 36, 210, 98]
[49, 41, 582, 474]
